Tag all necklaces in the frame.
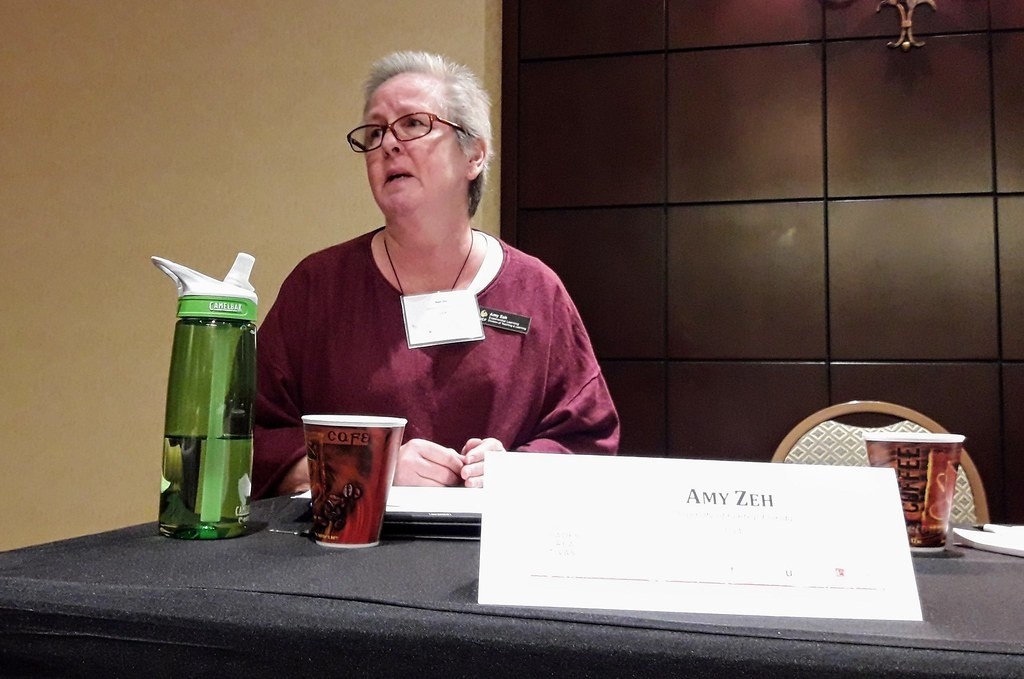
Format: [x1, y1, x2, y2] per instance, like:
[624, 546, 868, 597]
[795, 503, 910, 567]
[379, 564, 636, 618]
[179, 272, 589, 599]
[384, 232, 474, 295]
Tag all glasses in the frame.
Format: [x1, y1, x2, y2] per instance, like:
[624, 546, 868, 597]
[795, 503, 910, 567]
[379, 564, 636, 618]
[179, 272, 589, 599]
[347, 111, 479, 153]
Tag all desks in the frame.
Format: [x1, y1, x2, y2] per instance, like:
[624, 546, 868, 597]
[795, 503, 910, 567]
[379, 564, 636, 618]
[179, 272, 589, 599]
[0, 490, 1024, 679]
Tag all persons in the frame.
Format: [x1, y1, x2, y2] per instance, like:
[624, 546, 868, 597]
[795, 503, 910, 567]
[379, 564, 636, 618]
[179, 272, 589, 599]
[225, 51, 620, 500]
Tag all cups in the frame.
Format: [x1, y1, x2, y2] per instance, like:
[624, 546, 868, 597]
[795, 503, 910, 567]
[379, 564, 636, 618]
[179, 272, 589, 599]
[301, 414, 406, 549]
[863, 433, 964, 553]
[151, 253, 257, 540]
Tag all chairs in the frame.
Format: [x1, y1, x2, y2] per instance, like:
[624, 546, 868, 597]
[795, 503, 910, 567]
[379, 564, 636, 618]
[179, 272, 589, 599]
[770, 400, 991, 523]
